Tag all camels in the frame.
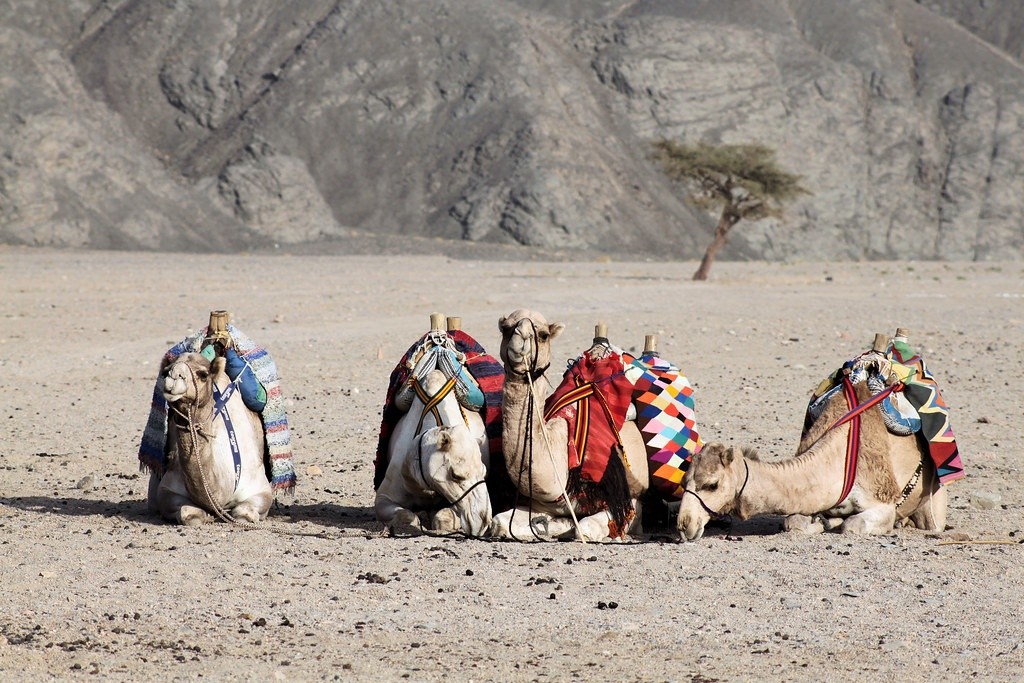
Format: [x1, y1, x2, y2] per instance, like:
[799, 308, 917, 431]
[372, 309, 707, 544]
[677, 326, 966, 546]
[138, 311, 296, 527]
